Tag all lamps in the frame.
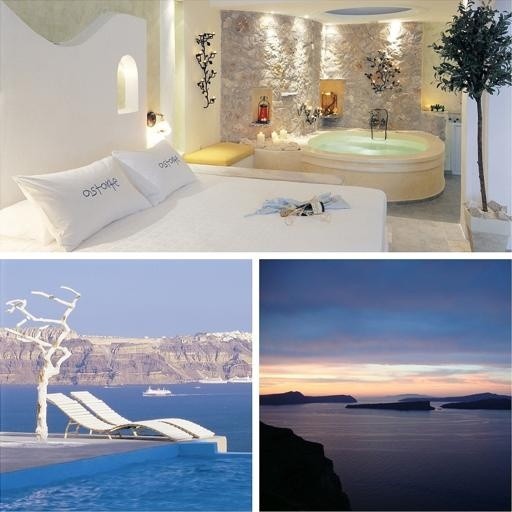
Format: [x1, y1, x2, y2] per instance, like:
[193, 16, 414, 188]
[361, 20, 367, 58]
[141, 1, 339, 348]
[147, 110, 173, 137]
[193, 28, 223, 109]
[296, 101, 324, 131]
[364, 50, 405, 93]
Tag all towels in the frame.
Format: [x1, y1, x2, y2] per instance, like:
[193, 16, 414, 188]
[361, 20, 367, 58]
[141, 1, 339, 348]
[247, 190, 352, 218]
[264, 140, 302, 152]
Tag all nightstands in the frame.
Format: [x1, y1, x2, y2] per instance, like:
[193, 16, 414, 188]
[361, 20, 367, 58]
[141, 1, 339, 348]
[182, 142, 254, 166]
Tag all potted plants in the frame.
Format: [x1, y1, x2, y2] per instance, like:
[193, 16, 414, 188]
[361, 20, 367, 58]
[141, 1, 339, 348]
[423, 0, 512, 251]
[431, 104, 443, 113]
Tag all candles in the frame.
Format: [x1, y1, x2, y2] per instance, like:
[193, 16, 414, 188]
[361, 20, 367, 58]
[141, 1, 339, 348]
[257, 128, 288, 143]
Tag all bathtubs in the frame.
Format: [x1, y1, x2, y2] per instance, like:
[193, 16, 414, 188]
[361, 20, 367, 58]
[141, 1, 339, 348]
[300, 128, 447, 203]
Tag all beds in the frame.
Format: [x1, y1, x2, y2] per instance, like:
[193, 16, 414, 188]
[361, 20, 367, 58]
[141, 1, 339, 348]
[0, 173, 389, 252]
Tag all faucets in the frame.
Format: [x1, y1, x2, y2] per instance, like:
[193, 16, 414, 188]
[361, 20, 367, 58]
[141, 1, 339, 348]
[376, 124, 380, 131]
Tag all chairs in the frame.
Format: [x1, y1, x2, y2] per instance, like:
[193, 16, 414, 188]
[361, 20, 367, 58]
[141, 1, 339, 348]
[70, 390, 215, 440]
[44, 392, 194, 441]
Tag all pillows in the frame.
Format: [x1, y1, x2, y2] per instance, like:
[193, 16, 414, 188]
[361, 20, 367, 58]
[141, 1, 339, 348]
[0, 195, 55, 248]
[9, 153, 152, 255]
[113, 137, 198, 207]
